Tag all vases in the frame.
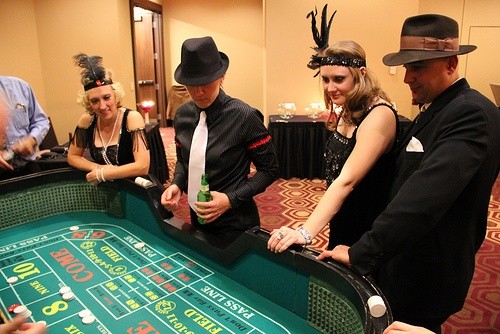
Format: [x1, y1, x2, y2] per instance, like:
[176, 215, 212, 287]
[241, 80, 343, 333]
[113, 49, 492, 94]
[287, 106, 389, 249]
[144, 112, 150, 124]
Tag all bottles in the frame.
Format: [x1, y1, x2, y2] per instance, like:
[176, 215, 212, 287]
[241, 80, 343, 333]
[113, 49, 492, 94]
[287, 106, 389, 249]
[197, 174, 211, 224]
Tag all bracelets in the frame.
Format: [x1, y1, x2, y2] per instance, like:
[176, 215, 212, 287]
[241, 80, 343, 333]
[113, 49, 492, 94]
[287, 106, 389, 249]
[296, 225, 312, 252]
[95, 167, 105, 183]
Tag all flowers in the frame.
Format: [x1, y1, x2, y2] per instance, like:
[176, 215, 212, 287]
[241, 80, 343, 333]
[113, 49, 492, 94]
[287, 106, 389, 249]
[136, 101, 155, 113]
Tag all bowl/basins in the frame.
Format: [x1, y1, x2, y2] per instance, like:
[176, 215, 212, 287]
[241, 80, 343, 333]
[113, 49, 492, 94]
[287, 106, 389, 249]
[278, 103, 296, 119]
[305, 103, 325, 119]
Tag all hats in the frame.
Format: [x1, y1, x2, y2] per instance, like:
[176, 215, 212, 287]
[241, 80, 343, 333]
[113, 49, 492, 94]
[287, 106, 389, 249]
[173, 36, 230, 87]
[382, 14, 477, 66]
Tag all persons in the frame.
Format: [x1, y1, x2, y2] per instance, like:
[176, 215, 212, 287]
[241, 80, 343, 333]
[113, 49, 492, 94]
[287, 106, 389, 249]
[1, 74, 52, 172]
[268, 3, 401, 254]
[317, 13, 500, 333]
[381, 320, 436, 333]
[160, 35, 280, 242]
[0, 314, 48, 334]
[67, 51, 151, 186]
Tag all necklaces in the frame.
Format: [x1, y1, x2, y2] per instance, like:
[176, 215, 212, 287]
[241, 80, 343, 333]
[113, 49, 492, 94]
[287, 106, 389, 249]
[95, 107, 120, 166]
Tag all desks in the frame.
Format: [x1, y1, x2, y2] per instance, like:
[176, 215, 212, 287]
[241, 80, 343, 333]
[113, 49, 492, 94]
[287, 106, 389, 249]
[268, 115, 410, 180]
[146, 123, 169, 184]
[0, 168, 393, 334]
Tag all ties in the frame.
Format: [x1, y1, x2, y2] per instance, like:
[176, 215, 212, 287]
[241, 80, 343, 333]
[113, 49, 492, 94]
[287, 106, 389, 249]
[187, 111, 208, 215]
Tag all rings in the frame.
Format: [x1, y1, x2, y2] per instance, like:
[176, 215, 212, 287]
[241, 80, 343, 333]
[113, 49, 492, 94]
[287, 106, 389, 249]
[277, 230, 284, 240]
